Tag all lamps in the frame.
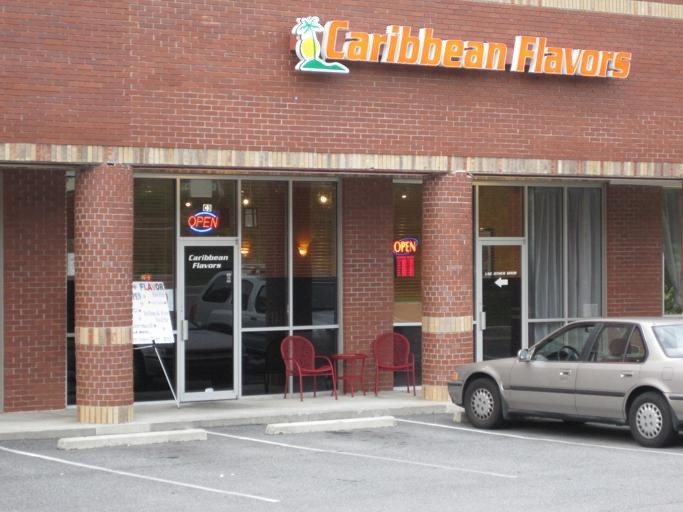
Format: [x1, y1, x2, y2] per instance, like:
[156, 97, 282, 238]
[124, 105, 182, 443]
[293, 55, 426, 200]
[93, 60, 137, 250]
[295, 238, 309, 257]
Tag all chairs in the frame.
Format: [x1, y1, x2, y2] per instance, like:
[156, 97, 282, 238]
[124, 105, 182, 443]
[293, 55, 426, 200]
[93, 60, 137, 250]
[601, 338, 635, 362]
[278, 334, 339, 402]
[371, 329, 418, 396]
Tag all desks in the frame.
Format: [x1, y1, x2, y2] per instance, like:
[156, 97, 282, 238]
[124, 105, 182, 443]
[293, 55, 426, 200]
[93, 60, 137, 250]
[329, 352, 368, 398]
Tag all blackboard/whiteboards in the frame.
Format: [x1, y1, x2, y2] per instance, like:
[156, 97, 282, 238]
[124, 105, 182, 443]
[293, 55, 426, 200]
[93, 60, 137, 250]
[132, 282, 175, 344]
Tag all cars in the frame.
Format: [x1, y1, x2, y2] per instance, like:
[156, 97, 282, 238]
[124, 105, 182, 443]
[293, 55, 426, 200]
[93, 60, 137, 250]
[444, 313, 682, 447]
[134, 306, 246, 392]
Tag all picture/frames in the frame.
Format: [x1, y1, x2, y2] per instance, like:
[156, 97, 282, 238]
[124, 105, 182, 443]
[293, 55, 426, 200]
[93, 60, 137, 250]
[478, 225, 494, 277]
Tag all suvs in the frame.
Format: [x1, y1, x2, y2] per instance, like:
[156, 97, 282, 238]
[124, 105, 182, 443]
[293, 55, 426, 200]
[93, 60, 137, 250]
[188, 269, 336, 372]
